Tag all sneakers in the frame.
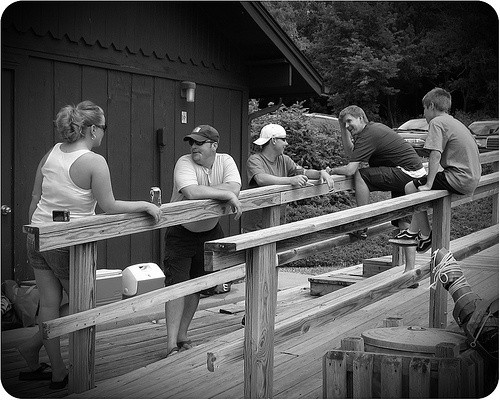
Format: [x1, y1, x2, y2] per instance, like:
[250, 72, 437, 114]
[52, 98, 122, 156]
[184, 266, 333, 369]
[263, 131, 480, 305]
[416, 230, 432, 254]
[388, 228, 418, 246]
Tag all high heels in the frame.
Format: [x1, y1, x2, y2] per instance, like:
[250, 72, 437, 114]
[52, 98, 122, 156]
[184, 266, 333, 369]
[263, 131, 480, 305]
[50, 372, 69, 389]
[19, 363, 52, 381]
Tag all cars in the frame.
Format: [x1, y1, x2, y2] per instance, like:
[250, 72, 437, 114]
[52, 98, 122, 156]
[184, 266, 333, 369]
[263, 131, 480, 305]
[395, 118, 430, 149]
[468, 120, 499, 151]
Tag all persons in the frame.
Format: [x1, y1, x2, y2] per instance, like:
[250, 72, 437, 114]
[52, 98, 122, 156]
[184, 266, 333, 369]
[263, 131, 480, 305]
[19, 100, 163, 391]
[163, 125, 242, 357]
[242, 124, 335, 234]
[388, 88, 482, 254]
[326, 106, 427, 288]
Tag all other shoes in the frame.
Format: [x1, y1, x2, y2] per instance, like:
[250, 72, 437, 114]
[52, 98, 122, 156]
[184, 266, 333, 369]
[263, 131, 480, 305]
[166, 347, 179, 358]
[349, 227, 368, 239]
[241, 315, 246, 327]
[177, 340, 193, 350]
[408, 283, 419, 289]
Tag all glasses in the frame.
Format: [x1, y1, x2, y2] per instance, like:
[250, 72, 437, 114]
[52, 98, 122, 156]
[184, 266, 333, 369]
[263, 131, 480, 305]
[274, 138, 287, 142]
[190, 140, 215, 146]
[88, 124, 107, 133]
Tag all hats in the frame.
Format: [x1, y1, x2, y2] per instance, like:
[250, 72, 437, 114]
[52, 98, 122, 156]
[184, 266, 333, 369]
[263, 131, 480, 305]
[253, 123, 286, 145]
[183, 125, 220, 143]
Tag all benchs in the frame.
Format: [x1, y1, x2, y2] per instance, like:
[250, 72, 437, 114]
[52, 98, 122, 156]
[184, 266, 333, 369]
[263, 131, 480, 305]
[21, 270, 124, 323]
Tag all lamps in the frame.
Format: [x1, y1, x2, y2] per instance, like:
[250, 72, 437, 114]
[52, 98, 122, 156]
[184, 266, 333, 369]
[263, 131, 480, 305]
[181, 81, 196, 102]
[121, 262, 164, 300]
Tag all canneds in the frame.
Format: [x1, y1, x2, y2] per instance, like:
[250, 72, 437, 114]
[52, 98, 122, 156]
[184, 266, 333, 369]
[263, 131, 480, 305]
[149, 186, 161, 207]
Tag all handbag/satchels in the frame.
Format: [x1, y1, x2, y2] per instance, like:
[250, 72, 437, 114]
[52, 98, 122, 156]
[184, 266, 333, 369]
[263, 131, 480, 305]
[1, 280, 40, 328]
